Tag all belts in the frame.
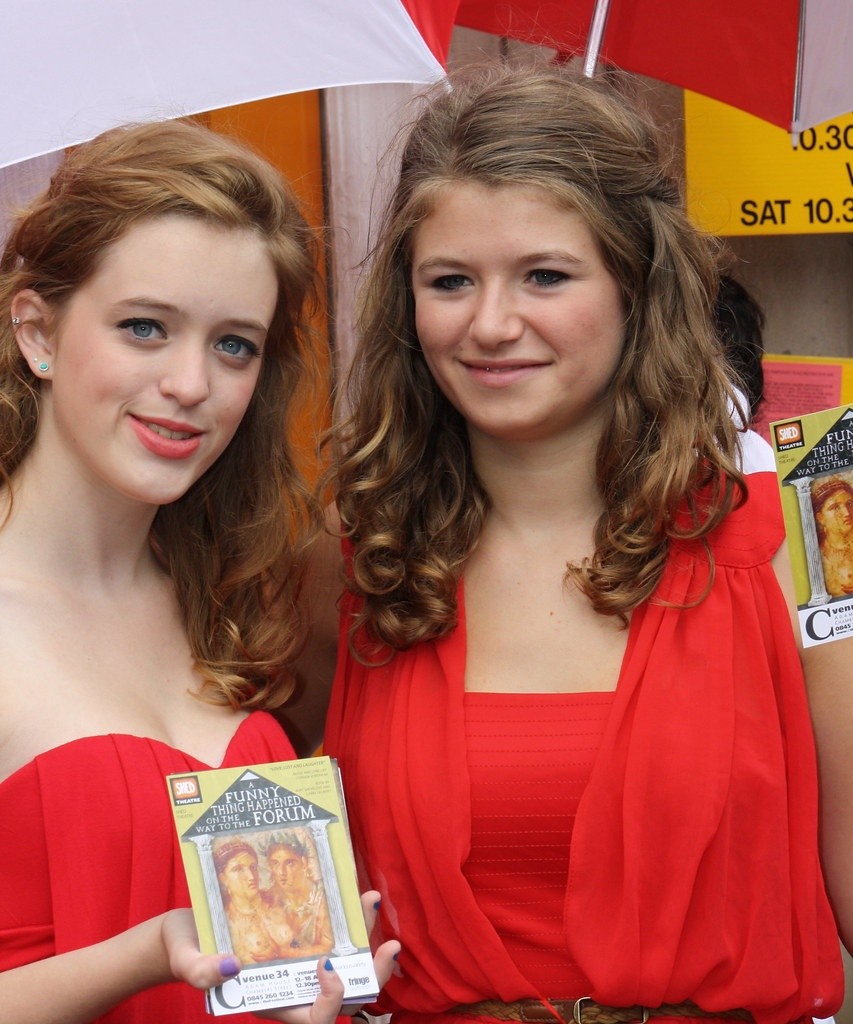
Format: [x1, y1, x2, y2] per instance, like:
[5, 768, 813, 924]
[455, 996, 741, 1024]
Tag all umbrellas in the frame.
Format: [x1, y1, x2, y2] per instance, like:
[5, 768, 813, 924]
[0, 1, 853, 169]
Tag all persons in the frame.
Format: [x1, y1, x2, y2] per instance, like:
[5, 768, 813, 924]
[0, 119, 403, 1024]
[320, 70, 853, 1024]
